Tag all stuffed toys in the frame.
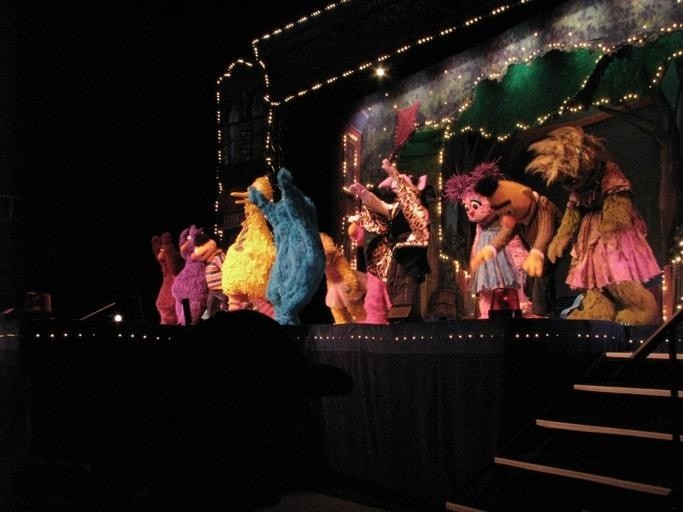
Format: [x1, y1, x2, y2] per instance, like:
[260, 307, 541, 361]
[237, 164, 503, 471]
[148, 123, 664, 326]
[147, 123, 664, 326]
